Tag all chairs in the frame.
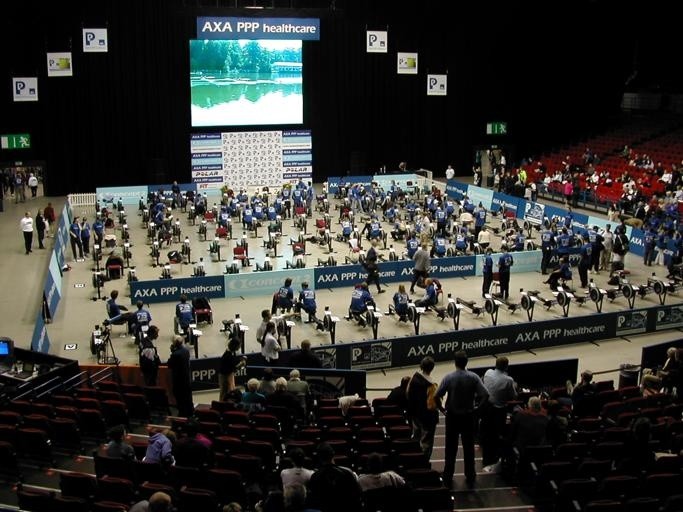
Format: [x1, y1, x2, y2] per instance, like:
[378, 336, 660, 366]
[506, 378, 683, 512]
[511, 118, 683, 221]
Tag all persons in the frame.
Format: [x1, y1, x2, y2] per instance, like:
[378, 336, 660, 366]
[1, 144, 683, 512]
